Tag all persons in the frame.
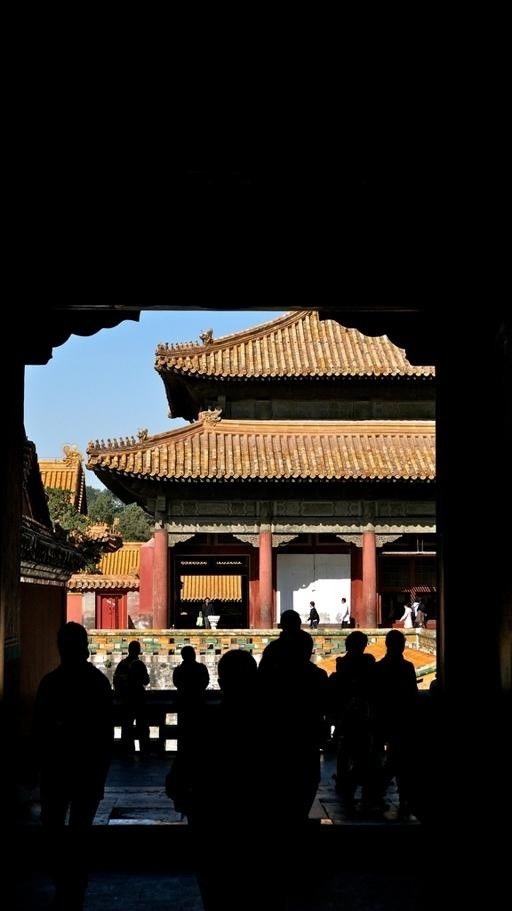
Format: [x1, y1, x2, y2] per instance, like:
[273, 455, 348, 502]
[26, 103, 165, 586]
[32, 620, 116, 911]
[306, 601, 320, 630]
[112, 641, 151, 754]
[398, 597, 429, 631]
[339, 598, 351, 629]
[201, 597, 216, 630]
[163, 607, 503, 907]
